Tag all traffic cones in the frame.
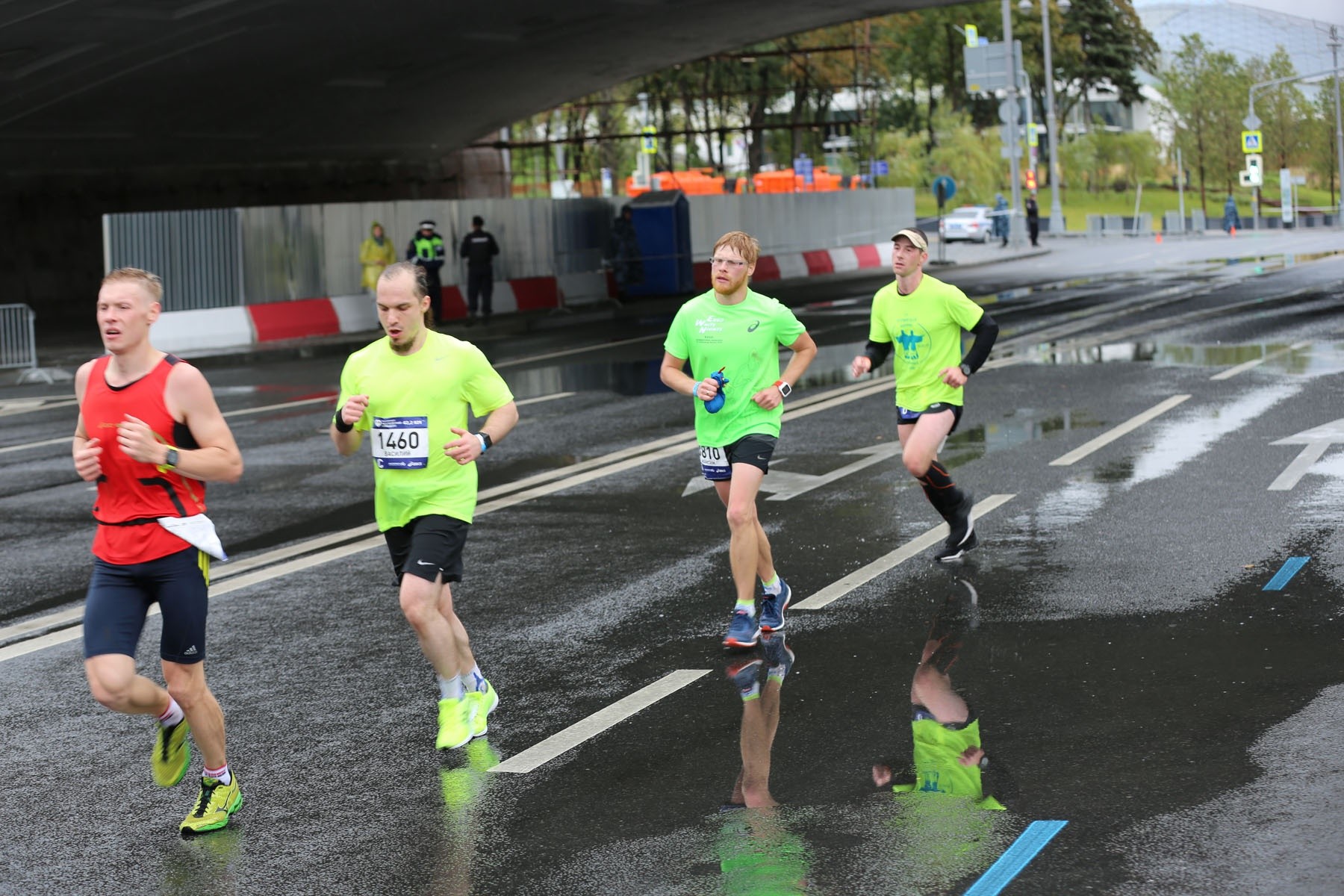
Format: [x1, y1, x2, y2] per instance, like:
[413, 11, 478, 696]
[1230, 226, 1237, 236]
[1155, 232, 1162, 243]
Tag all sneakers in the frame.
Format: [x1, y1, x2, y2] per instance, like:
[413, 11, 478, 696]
[727, 659, 763, 693]
[722, 609, 761, 647]
[759, 631, 795, 675]
[152, 716, 191, 788]
[759, 578, 791, 630]
[179, 770, 243, 834]
[464, 680, 499, 737]
[436, 695, 473, 750]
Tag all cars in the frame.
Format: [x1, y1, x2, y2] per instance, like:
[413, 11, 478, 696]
[937, 204, 998, 244]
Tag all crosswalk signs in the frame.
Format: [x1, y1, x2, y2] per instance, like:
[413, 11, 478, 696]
[641, 125, 658, 155]
[1026, 122, 1040, 147]
[1240, 130, 1264, 154]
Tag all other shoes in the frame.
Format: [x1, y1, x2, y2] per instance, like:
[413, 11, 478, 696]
[947, 576, 983, 630]
[439, 735, 500, 808]
[945, 495, 975, 548]
[934, 530, 980, 562]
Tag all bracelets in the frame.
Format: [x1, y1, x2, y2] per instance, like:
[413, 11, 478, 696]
[693, 382, 702, 397]
[335, 408, 353, 434]
[475, 433, 487, 455]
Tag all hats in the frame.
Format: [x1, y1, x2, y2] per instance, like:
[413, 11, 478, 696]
[420, 220, 436, 231]
[890, 229, 928, 253]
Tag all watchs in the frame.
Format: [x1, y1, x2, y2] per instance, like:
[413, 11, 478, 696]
[959, 364, 973, 377]
[475, 432, 492, 448]
[774, 380, 792, 397]
[158, 445, 178, 475]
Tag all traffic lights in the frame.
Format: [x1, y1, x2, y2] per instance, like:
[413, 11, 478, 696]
[1239, 153, 1264, 188]
[1025, 168, 1038, 191]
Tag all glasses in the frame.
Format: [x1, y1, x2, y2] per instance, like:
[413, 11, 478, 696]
[710, 256, 748, 268]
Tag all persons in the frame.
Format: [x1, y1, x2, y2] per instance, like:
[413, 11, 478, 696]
[1026, 191, 1039, 246]
[872, 580, 1009, 808]
[330, 262, 527, 750]
[72, 268, 245, 835]
[660, 230, 818, 647]
[359, 223, 399, 332]
[718, 633, 794, 811]
[849, 227, 999, 560]
[407, 220, 446, 324]
[461, 215, 500, 327]
